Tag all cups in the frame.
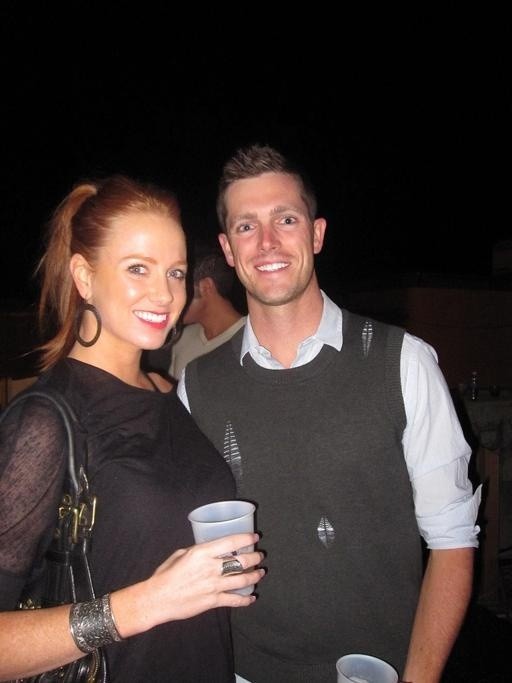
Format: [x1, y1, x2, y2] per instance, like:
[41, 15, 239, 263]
[187, 500, 257, 601]
[336, 653, 399, 683]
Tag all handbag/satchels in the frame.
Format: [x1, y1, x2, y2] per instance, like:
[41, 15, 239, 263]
[0, 388, 108, 683]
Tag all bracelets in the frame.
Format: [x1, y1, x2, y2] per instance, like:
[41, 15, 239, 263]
[68, 589, 124, 656]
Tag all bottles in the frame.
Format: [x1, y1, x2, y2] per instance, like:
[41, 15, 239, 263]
[468, 370, 482, 402]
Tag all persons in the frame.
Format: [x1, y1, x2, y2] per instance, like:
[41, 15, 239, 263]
[160, 241, 252, 385]
[171, 142, 483, 681]
[2, 171, 269, 681]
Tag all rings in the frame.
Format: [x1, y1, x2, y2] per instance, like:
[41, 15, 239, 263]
[221, 553, 244, 577]
